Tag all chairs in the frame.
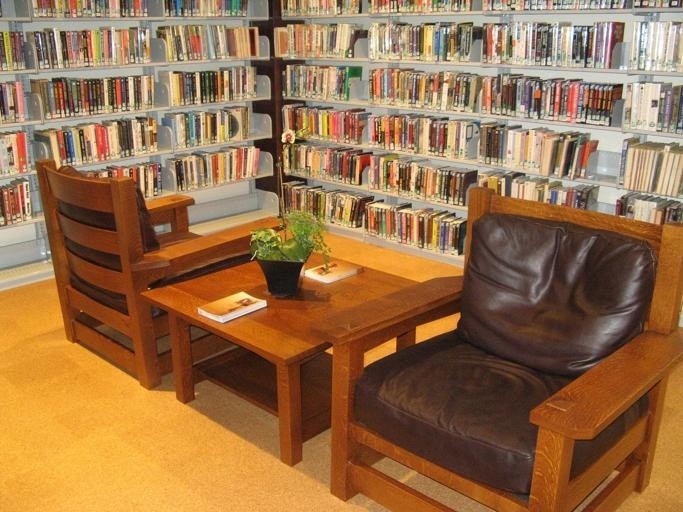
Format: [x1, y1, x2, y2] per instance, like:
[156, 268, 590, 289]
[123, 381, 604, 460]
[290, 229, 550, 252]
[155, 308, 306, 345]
[313, 187, 682, 510]
[37, 160, 282, 390]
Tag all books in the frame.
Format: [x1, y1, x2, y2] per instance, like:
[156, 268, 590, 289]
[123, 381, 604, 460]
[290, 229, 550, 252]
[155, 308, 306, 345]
[273, 0, 361, 59]
[278, 63, 370, 145]
[280, 140, 373, 229]
[198, 291, 267, 323]
[364, 113, 483, 255]
[1, 0, 261, 71]
[157, 65, 260, 191]
[478, 122, 683, 226]
[304, 260, 365, 285]
[366, 0, 682, 134]
[0, 75, 163, 226]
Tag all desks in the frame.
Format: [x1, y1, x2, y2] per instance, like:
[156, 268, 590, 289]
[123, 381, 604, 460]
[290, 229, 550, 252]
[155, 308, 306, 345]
[140, 252, 421, 467]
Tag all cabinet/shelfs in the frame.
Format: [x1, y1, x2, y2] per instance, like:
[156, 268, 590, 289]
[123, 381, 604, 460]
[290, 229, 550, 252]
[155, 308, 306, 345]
[270, 0, 682, 268]
[1, 1, 269, 248]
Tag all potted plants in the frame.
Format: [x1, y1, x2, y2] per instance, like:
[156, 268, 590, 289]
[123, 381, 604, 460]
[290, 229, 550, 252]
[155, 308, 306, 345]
[250, 209, 326, 297]
